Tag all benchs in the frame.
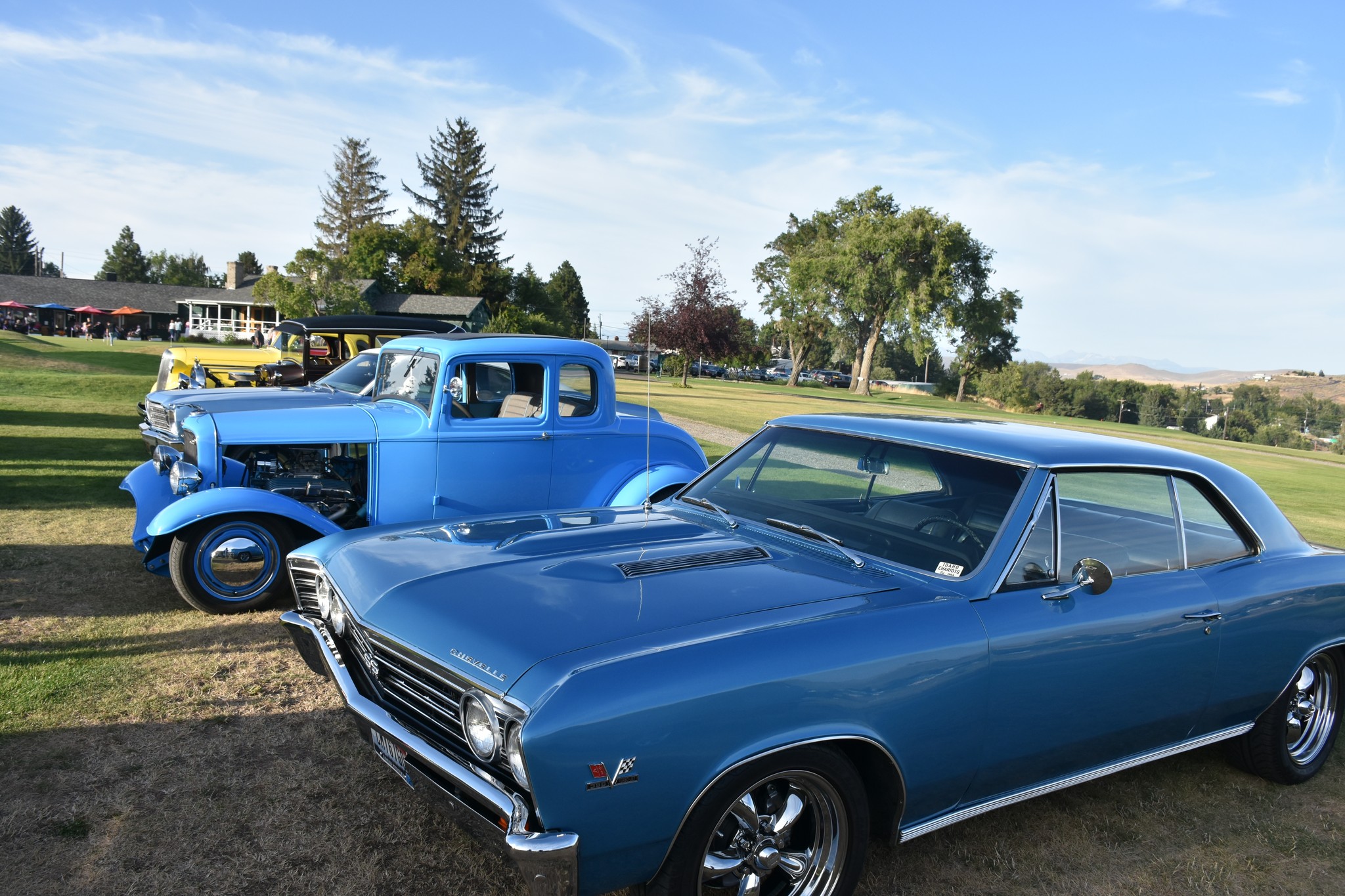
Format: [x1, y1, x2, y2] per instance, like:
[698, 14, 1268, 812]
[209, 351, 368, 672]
[965, 495, 1245, 570]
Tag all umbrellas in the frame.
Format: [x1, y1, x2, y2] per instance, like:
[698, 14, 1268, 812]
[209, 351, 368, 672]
[0, 300, 31, 314]
[109, 306, 143, 315]
[34, 303, 72, 322]
[72, 306, 108, 325]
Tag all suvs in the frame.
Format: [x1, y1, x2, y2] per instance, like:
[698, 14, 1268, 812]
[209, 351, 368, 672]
[609, 353, 670, 374]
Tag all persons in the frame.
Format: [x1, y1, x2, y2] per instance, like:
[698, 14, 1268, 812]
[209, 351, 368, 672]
[185, 320, 190, 338]
[26, 313, 35, 332]
[0, 308, 22, 333]
[86, 317, 93, 342]
[74, 321, 141, 339]
[168, 317, 183, 342]
[72, 316, 75, 321]
[106, 319, 121, 346]
[267, 326, 276, 349]
[254, 324, 264, 349]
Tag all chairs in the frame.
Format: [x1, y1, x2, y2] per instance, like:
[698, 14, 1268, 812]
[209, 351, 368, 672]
[862, 500, 957, 539]
[498, 392, 589, 419]
[1007, 527, 1129, 583]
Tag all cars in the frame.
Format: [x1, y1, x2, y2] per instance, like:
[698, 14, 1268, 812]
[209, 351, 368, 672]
[137, 313, 584, 432]
[687, 359, 853, 388]
[116, 332, 711, 616]
[278, 411, 1344, 896]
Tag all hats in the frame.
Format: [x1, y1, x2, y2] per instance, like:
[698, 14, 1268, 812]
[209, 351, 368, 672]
[137, 325, 140, 328]
[270, 324, 276, 328]
[29, 313, 32, 316]
[177, 317, 180, 320]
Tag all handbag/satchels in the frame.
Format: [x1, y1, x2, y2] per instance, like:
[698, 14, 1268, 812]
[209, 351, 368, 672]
[251, 336, 255, 343]
[251, 336, 255, 342]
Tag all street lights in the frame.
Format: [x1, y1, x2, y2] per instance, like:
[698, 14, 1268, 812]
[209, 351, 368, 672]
[924, 352, 932, 383]
